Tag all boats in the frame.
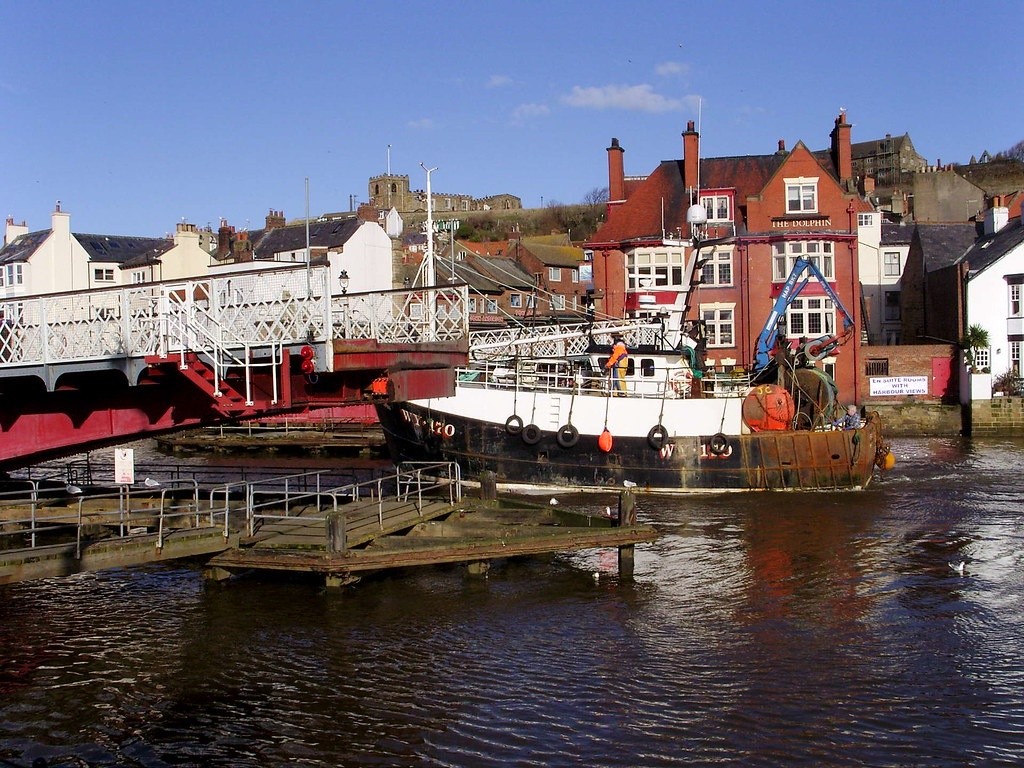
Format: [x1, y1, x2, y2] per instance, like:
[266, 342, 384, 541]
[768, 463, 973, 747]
[372, 97, 882, 495]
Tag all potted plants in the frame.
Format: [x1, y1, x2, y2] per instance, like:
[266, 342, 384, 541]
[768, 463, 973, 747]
[949, 325, 992, 373]
[993, 368, 1024, 396]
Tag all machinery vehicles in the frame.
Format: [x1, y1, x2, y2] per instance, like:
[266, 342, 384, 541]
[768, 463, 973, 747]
[743, 253, 856, 424]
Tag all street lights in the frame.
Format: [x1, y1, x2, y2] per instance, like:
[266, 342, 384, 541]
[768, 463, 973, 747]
[420, 161, 439, 340]
[442, 218, 461, 318]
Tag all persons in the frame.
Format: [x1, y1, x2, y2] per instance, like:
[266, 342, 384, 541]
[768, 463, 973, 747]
[832, 405, 860, 430]
[601, 332, 629, 397]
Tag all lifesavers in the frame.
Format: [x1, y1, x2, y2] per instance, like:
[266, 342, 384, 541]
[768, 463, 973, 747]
[709, 433, 728, 453]
[522, 424, 540, 444]
[557, 425, 580, 448]
[504, 415, 524, 433]
[648, 426, 670, 451]
